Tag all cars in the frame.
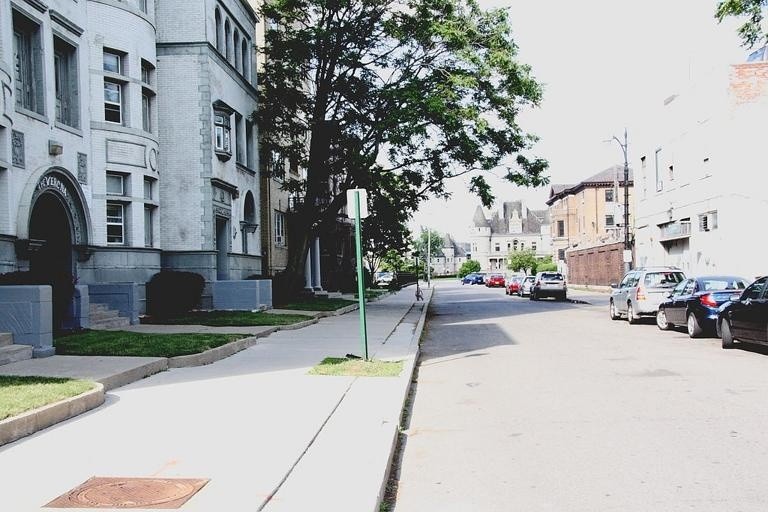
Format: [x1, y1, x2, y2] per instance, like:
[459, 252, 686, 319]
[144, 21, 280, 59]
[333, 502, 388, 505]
[609, 266, 686, 325]
[715, 276, 767, 349]
[655, 275, 752, 338]
[460, 274, 482, 285]
[484, 272, 566, 301]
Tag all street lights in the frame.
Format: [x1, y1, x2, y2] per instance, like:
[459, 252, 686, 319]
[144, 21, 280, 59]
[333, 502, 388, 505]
[601, 127, 630, 275]
